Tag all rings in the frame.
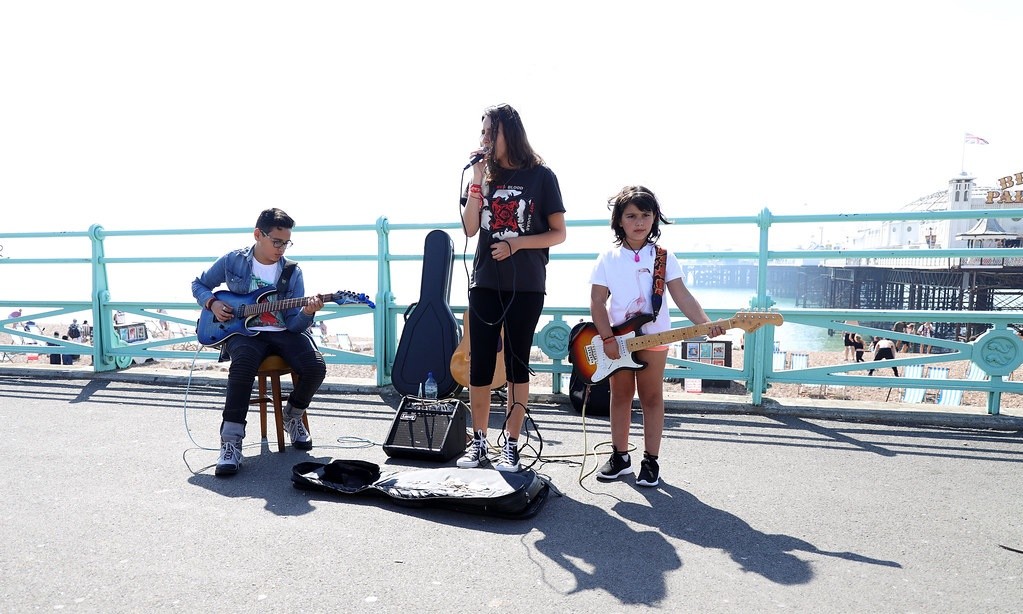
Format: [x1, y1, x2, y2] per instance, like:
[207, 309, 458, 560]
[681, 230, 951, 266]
[223, 306, 227, 310]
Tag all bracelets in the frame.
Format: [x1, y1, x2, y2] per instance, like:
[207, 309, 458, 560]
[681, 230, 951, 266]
[603, 339, 616, 345]
[603, 335, 615, 341]
[207, 298, 219, 311]
[469, 184, 483, 209]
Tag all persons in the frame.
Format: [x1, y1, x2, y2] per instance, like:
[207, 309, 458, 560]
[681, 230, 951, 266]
[54, 318, 91, 340]
[589, 185, 726, 486]
[455, 104, 567, 473]
[841, 318, 935, 377]
[192, 208, 327, 479]
[7, 309, 24, 330]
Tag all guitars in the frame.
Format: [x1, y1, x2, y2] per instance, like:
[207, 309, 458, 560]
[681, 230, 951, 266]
[568, 308, 784, 385]
[195, 286, 375, 348]
[450, 308, 507, 390]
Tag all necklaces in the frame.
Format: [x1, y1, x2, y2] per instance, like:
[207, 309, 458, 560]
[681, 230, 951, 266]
[625, 236, 648, 263]
[499, 168, 518, 188]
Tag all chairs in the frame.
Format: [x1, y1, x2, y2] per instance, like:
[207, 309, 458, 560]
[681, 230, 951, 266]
[143, 319, 197, 350]
[773, 338, 988, 405]
[0, 323, 48, 364]
[312, 326, 364, 351]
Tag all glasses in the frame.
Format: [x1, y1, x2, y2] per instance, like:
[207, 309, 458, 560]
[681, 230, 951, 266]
[261, 230, 293, 249]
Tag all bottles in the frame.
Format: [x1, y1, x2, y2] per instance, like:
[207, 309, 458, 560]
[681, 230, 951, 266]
[425, 372, 437, 400]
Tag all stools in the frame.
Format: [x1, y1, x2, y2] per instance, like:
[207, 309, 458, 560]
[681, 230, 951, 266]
[250, 357, 311, 456]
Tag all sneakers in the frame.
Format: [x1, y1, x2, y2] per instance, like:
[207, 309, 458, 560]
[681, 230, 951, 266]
[283, 409, 312, 449]
[496, 431, 521, 472]
[457, 428, 488, 467]
[596, 445, 634, 479]
[636, 451, 660, 486]
[215, 433, 244, 474]
